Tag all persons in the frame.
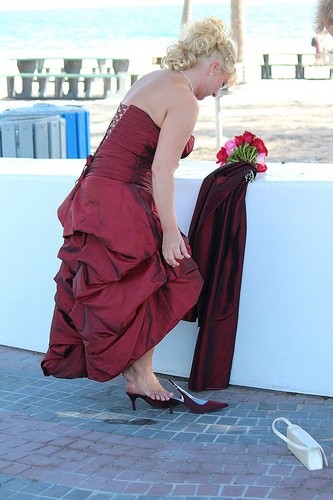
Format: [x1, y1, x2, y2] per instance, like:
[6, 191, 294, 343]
[40, 17, 239, 411]
[311, 36, 323, 61]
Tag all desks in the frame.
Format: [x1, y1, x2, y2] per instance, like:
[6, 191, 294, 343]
[15, 59, 129, 90]
[0, 157, 333, 398]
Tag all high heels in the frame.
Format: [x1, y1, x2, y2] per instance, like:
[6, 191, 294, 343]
[168, 378, 229, 414]
[126, 380, 180, 411]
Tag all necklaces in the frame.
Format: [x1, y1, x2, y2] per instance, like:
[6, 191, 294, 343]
[181, 70, 194, 92]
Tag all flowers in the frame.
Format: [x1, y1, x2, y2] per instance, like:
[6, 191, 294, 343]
[216, 131, 268, 183]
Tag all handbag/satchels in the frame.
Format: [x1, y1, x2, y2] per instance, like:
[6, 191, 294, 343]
[272, 417, 328, 470]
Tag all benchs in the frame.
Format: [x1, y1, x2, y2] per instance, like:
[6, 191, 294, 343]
[34, 67, 110, 92]
[0, 74, 138, 100]
[260, 52, 333, 80]
